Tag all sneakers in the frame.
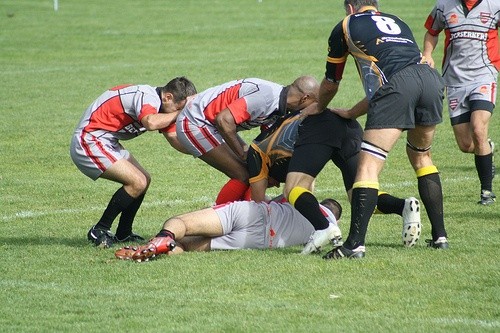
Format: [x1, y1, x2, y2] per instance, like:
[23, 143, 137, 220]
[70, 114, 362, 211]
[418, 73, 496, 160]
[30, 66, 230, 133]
[403, 197, 422, 248]
[322, 245, 366, 258]
[114, 233, 145, 242]
[87, 225, 113, 248]
[115, 236, 176, 262]
[478, 191, 496, 205]
[302, 223, 343, 254]
[487, 138, 495, 179]
[425, 237, 448, 250]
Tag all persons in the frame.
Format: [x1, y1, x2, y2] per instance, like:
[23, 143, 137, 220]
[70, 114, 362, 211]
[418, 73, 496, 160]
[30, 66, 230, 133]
[69, 75, 198, 249]
[299, 0, 450, 260]
[419, 0, 500, 206]
[160, 75, 319, 206]
[113, 197, 343, 264]
[245, 106, 422, 257]
